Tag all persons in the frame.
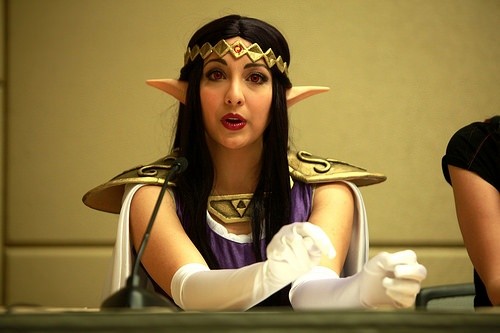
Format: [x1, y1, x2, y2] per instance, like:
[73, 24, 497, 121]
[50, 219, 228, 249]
[441, 116, 500, 309]
[83, 15, 427, 311]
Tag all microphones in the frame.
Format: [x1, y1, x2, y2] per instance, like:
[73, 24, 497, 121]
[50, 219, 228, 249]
[126, 156, 189, 288]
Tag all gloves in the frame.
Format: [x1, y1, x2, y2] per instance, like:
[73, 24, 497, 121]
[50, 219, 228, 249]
[169, 221, 336, 312]
[287, 248, 427, 311]
